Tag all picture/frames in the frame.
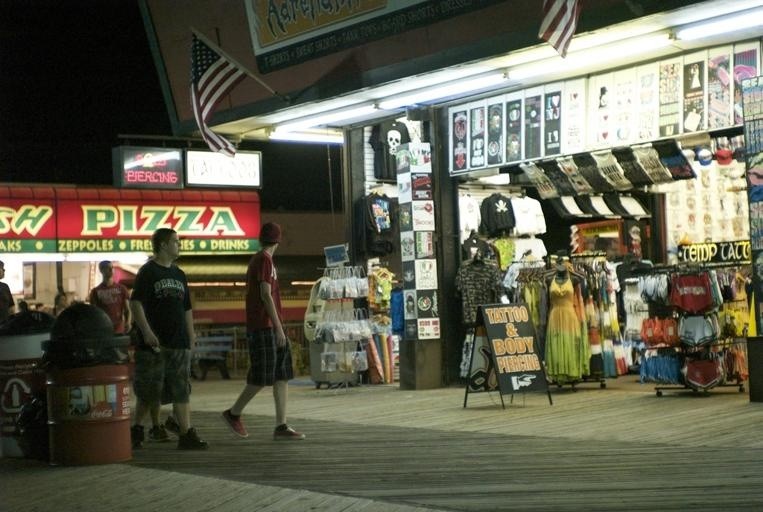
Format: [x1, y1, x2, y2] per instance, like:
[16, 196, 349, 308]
[23, 262, 36, 300]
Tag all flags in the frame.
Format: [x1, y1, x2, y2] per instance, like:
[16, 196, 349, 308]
[537, 0, 584, 58]
[189, 31, 247, 159]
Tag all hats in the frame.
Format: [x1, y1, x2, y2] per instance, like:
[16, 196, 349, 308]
[261, 223, 281, 242]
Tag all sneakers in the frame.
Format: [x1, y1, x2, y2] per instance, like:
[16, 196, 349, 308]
[221, 411, 248, 437]
[274, 427, 305, 439]
[131, 416, 208, 449]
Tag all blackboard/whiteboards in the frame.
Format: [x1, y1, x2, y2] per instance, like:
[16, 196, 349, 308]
[481, 302, 549, 395]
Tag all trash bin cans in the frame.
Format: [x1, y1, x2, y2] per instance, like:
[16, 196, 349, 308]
[0, 303, 133, 466]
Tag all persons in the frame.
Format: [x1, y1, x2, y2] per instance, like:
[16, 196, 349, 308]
[130, 228, 208, 450]
[89, 260, 133, 337]
[221, 222, 306, 442]
[149, 396, 182, 443]
[543, 265, 591, 385]
[690, 64, 701, 90]
[0, 260, 67, 324]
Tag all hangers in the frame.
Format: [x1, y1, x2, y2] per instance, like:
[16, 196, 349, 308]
[637, 262, 749, 363]
[515, 250, 608, 290]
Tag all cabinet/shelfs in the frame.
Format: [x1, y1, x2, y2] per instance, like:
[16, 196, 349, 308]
[571, 219, 653, 265]
